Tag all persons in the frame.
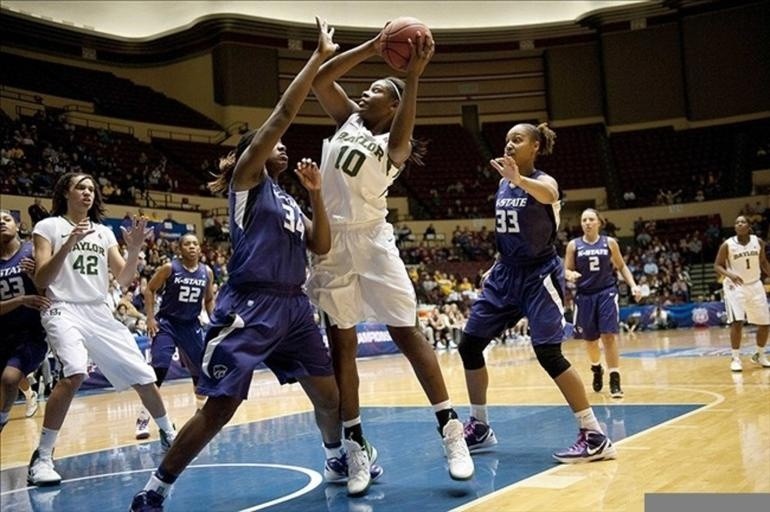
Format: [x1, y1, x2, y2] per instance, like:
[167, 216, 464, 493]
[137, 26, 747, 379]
[564, 208, 643, 396]
[1, 210, 51, 431]
[310, 19, 475, 498]
[1, 110, 769, 351]
[136, 233, 216, 440]
[462, 122, 618, 465]
[34, 358, 54, 401]
[26, 173, 179, 486]
[713, 214, 769, 372]
[128, 15, 384, 512]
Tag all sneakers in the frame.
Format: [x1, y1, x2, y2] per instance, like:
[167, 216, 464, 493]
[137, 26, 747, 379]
[28, 488, 61, 512]
[322, 438, 383, 497]
[159, 424, 178, 454]
[435, 419, 475, 480]
[731, 356, 742, 371]
[25, 390, 38, 418]
[463, 415, 497, 451]
[26, 446, 62, 487]
[750, 351, 770, 368]
[128, 489, 164, 512]
[609, 371, 624, 397]
[134, 414, 150, 439]
[591, 364, 604, 392]
[552, 422, 616, 462]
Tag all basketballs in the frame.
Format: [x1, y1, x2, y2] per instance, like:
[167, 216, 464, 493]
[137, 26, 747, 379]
[380, 16, 433, 72]
